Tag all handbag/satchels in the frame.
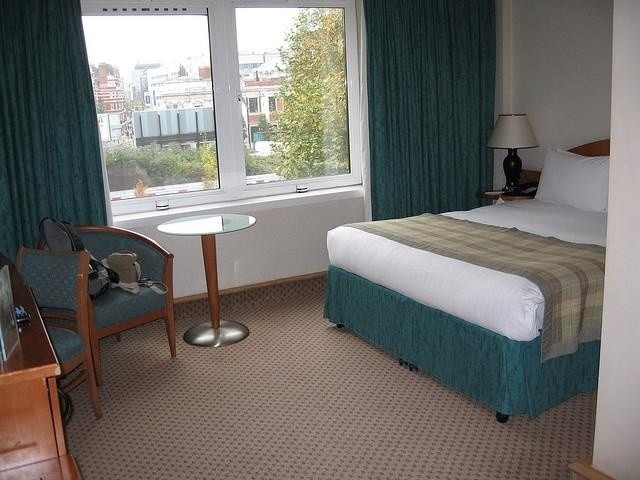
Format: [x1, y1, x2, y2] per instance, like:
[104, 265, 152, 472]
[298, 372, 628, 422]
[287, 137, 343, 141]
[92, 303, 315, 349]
[107, 250, 142, 283]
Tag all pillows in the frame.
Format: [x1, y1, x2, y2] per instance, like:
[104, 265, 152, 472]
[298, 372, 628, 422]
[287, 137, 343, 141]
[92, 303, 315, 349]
[534, 146, 610, 214]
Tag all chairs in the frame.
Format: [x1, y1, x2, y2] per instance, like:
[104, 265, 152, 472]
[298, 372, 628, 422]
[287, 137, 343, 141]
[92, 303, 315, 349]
[18, 224, 175, 421]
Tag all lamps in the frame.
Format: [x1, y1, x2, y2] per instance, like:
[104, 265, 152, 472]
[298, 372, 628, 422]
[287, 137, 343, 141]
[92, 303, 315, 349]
[485, 112, 540, 195]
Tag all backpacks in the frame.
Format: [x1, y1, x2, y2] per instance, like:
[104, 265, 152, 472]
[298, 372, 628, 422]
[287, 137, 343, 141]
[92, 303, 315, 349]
[38, 216, 120, 299]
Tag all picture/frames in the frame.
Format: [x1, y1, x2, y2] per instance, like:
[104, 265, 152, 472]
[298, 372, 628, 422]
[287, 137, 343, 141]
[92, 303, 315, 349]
[0, 264, 21, 363]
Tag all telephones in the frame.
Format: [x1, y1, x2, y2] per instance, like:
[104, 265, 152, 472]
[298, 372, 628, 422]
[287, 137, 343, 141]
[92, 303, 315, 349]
[516, 182, 538, 196]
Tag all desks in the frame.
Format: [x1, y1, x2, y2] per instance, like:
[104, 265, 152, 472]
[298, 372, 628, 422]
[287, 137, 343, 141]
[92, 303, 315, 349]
[156, 212, 258, 346]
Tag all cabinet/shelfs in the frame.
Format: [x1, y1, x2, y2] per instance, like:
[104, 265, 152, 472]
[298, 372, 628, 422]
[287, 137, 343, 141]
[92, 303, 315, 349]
[0, 252, 84, 479]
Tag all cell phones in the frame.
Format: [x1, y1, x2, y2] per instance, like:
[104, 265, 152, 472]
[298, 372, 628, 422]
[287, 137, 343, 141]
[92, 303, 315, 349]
[15, 304, 32, 323]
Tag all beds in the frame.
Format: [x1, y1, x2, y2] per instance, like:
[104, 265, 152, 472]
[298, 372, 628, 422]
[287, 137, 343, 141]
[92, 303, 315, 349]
[325, 135, 612, 424]
